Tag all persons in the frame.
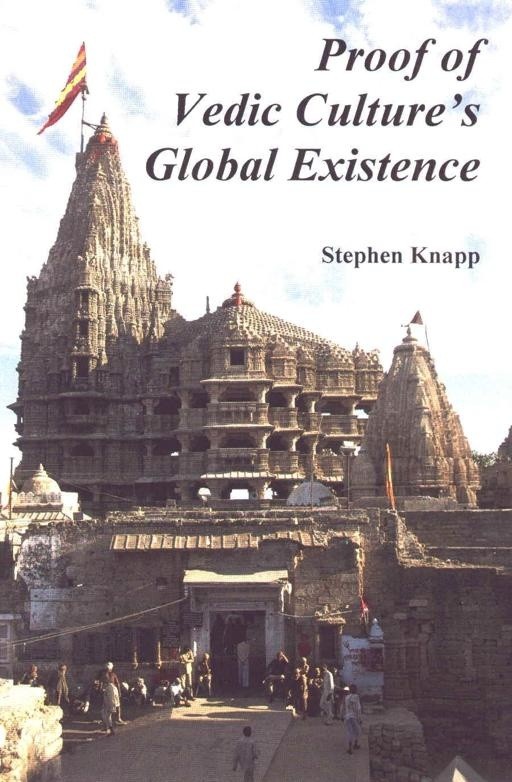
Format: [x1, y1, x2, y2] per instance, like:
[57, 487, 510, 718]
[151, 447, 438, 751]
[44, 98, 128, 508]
[258, 650, 363, 754]
[233, 725, 260, 781]
[92, 680, 122, 736]
[131, 646, 212, 708]
[55, 663, 72, 723]
[100, 660, 127, 727]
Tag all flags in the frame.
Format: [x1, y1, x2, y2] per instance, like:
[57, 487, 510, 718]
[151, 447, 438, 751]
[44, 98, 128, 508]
[36, 40, 89, 134]
